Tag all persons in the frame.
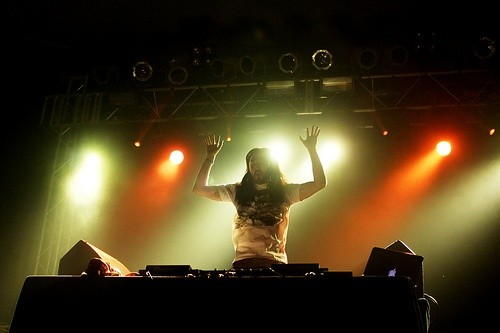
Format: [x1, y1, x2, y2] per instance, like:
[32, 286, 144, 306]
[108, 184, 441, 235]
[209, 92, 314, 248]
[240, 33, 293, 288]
[191, 125, 327, 269]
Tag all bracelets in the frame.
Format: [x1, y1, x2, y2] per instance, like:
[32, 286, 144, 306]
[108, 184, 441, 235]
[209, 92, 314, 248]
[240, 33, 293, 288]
[206, 158, 214, 165]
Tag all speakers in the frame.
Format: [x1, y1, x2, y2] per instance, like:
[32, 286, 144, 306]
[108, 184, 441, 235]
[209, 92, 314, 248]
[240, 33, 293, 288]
[361, 238, 424, 297]
[57, 240, 131, 276]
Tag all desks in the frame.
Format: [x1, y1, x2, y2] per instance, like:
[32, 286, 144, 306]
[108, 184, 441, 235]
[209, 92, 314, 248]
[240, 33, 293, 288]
[9, 276, 427, 333]
